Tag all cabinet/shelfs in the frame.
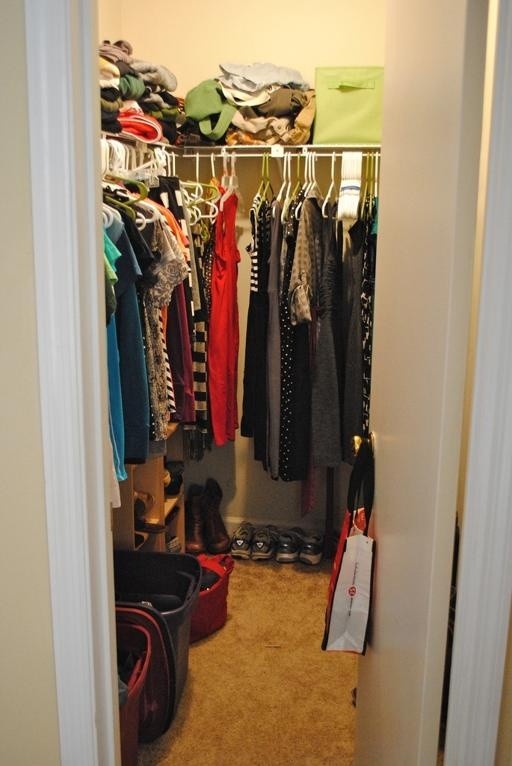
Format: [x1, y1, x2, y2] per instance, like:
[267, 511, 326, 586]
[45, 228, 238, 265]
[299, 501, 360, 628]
[111, 422, 186, 555]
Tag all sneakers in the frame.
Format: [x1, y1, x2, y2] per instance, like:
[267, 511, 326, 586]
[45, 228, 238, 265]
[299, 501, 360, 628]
[231, 521, 325, 566]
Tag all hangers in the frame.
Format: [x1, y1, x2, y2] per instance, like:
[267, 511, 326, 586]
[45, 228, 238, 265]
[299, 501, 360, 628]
[102, 138, 380, 232]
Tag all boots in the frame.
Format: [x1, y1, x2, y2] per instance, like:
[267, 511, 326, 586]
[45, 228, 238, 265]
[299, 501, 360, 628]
[185, 483, 207, 555]
[201, 478, 230, 553]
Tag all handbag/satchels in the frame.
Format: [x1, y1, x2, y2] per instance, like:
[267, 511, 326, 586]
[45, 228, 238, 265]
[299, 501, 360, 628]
[191, 553, 234, 644]
[321, 507, 376, 656]
